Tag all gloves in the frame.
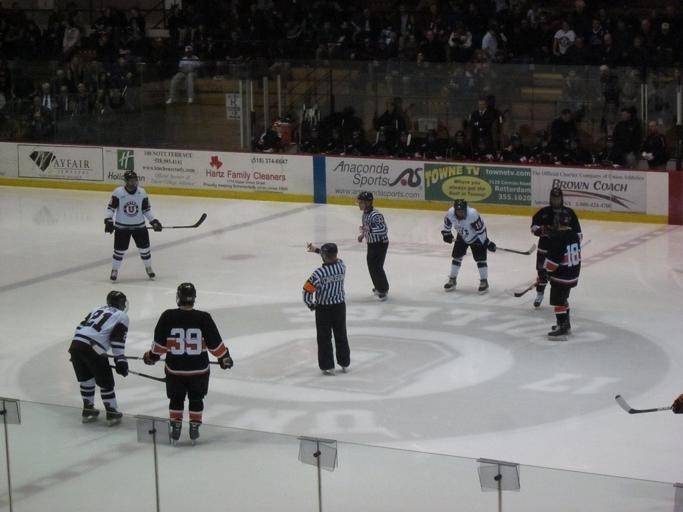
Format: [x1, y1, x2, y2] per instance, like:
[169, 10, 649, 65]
[487, 241, 496, 253]
[104, 218, 113, 234]
[149, 219, 162, 232]
[443, 234, 454, 244]
[114, 354, 129, 377]
[143, 350, 160, 366]
[218, 347, 233, 369]
[536, 275, 548, 292]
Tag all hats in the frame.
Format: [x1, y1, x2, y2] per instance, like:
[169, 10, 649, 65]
[320, 242, 338, 254]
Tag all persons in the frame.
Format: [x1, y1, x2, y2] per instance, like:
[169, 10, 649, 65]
[596, 33, 621, 65]
[640, 121, 669, 168]
[476, 135, 498, 164]
[302, 239, 351, 374]
[659, 3, 681, 23]
[612, 19, 632, 37]
[537, 213, 581, 340]
[640, 18, 654, 44]
[471, 99, 501, 135]
[440, 199, 496, 293]
[448, 24, 473, 64]
[1, 1, 152, 138]
[397, 25, 422, 61]
[419, 30, 442, 66]
[373, 97, 405, 131]
[158, 0, 316, 70]
[559, 134, 592, 167]
[67, 291, 131, 421]
[608, 136, 636, 168]
[426, 4, 444, 29]
[509, 130, 530, 165]
[567, 35, 592, 67]
[104, 172, 163, 281]
[525, 3, 544, 27]
[143, 283, 235, 440]
[168, 46, 201, 105]
[359, 8, 378, 33]
[591, 137, 608, 165]
[448, 130, 474, 162]
[373, 130, 398, 158]
[624, 36, 649, 70]
[397, 4, 415, 31]
[356, 191, 391, 296]
[529, 187, 583, 307]
[656, 23, 680, 61]
[326, 128, 345, 155]
[530, 130, 554, 164]
[346, 129, 372, 158]
[415, 129, 441, 160]
[481, 24, 499, 64]
[377, 22, 396, 60]
[552, 22, 576, 57]
[549, 109, 580, 135]
[315, 22, 336, 57]
[299, 127, 325, 153]
[612, 106, 642, 145]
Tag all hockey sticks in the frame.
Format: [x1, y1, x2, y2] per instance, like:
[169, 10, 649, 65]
[114, 213, 208, 231]
[92, 352, 221, 369]
[451, 237, 537, 255]
[502, 280, 538, 298]
[614, 395, 674, 414]
[109, 364, 166, 386]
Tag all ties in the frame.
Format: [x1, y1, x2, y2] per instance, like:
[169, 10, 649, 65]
[44, 96, 48, 106]
[480, 111, 483, 117]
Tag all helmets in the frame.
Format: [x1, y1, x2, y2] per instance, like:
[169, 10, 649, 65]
[106, 291, 126, 311]
[356, 191, 374, 202]
[175, 282, 197, 307]
[549, 187, 564, 208]
[124, 171, 139, 190]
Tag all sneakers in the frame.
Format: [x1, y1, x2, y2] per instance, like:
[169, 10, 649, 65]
[82, 405, 99, 416]
[548, 322, 568, 335]
[533, 292, 544, 307]
[110, 269, 118, 280]
[188, 98, 194, 104]
[478, 279, 488, 291]
[145, 267, 155, 277]
[189, 421, 200, 440]
[165, 98, 176, 105]
[552, 319, 571, 331]
[105, 410, 122, 420]
[170, 421, 182, 440]
[444, 277, 456, 289]
